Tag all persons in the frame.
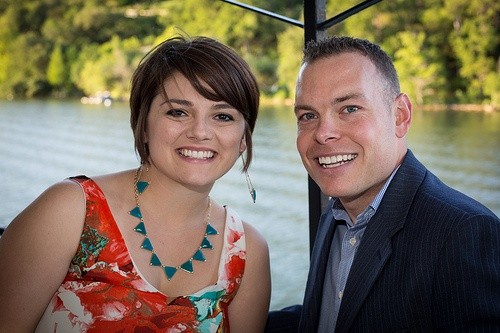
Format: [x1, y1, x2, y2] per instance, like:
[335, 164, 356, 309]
[0, 36, 271, 333]
[293, 36, 500, 333]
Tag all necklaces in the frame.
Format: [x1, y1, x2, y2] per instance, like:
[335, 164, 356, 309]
[128, 167, 220, 280]
